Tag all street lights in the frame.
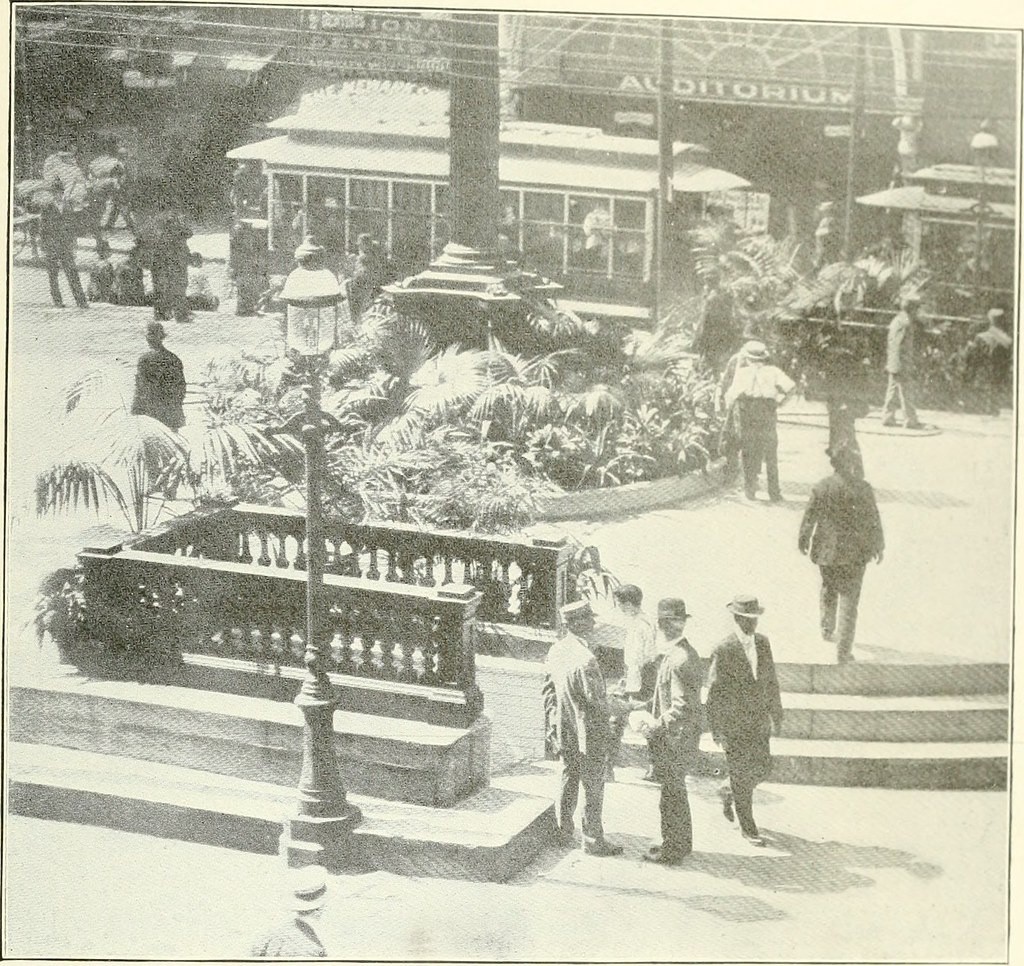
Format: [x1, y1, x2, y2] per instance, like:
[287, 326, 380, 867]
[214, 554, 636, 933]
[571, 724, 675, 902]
[968, 127, 1003, 323]
[264, 231, 366, 841]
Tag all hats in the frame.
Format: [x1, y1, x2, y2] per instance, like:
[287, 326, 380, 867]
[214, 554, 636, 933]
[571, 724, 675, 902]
[741, 340, 769, 359]
[143, 321, 167, 340]
[658, 597, 691, 620]
[559, 600, 598, 624]
[614, 585, 643, 607]
[727, 594, 765, 618]
[827, 439, 861, 465]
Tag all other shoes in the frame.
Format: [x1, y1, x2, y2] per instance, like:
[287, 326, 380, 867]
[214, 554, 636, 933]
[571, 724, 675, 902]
[742, 828, 766, 847]
[717, 786, 735, 822]
[587, 841, 623, 857]
[641, 845, 683, 866]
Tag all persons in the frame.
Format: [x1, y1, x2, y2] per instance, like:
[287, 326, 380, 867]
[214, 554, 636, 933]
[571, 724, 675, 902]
[629, 598, 703, 865]
[816, 324, 870, 480]
[602, 584, 661, 785]
[717, 340, 795, 503]
[581, 196, 616, 254]
[707, 595, 784, 848]
[541, 599, 623, 858]
[881, 293, 935, 430]
[798, 437, 886, 660]
[972, 307, 1013, 417]
[15, 136, 383, 326]
[129, 321, 187, 433]
